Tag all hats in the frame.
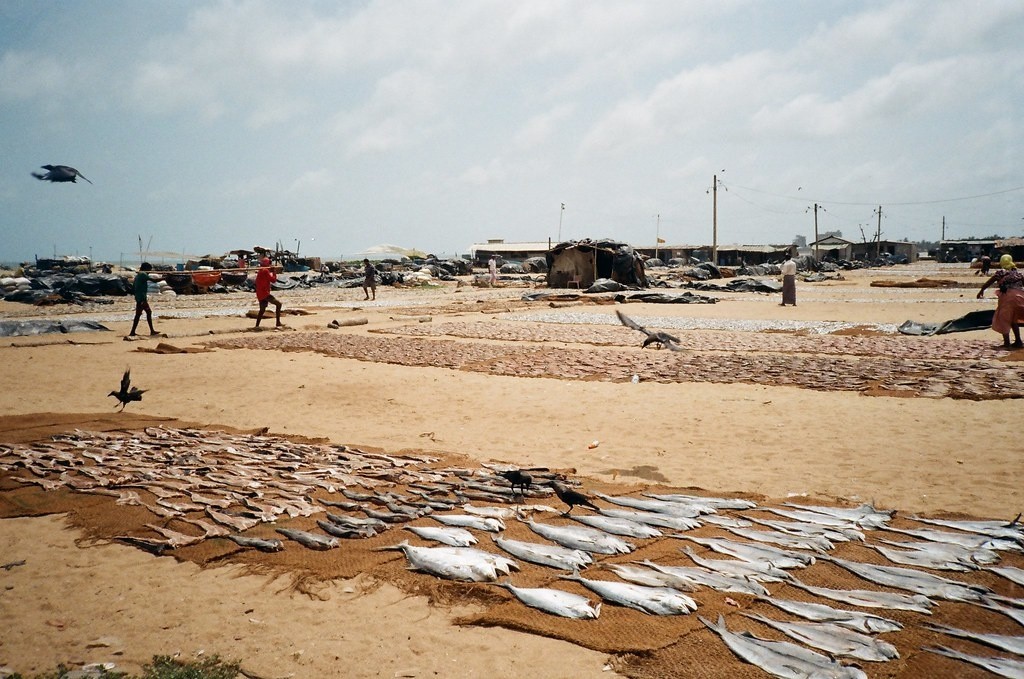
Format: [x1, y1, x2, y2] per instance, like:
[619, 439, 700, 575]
[260, 257, 270, 264]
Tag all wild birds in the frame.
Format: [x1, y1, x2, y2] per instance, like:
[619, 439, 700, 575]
[107, 365, 151, 413]
[30, 163, 94, 186]
[494, 468, 533, 497]
[616, 309, 691, 355]
[538, 480, 601, 518]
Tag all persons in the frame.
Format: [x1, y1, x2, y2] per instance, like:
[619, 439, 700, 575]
[488, 256, 496, 285]
[254, 257, 286, 330]
[130, 263, 167, 335]
[981, 255, 991, 273]
[977, 254, 1024, 347]
[362, 259, 377, 301]
[15, 262, 28, 279]
[101, 264, 112, 273]
[778, 254, 797, 306]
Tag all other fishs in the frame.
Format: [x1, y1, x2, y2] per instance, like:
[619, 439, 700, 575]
[0, 310, 1024, 679]
